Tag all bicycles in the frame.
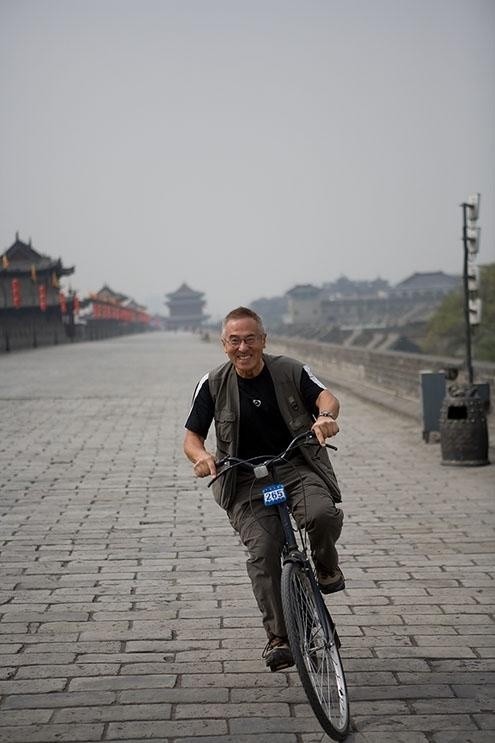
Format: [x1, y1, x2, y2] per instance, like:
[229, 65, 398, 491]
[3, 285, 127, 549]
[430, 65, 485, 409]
[207, 430, 350, 742]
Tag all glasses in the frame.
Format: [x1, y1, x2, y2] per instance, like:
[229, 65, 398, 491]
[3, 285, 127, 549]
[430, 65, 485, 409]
[223, 335, 261, 345]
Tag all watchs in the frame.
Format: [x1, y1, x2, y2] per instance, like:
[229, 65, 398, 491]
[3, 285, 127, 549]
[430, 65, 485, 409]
[317, 410, 336, 421]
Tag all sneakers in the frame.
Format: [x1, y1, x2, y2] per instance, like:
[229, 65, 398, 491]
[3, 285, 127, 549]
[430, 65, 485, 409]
[316, 560, 345, 594]
[263, 638, 293, 667]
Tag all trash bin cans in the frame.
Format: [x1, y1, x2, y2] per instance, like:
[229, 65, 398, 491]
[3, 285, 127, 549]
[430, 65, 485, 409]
[437, 383, 490, 467]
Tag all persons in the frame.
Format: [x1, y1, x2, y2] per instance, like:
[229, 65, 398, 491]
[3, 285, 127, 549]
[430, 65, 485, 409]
[183, 305, 348, 668]
